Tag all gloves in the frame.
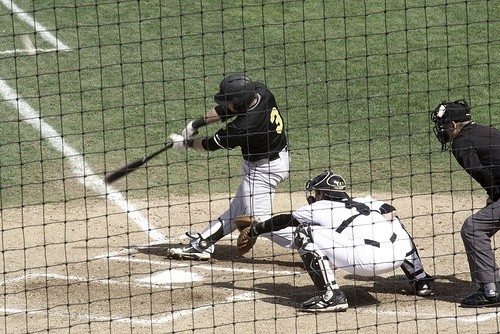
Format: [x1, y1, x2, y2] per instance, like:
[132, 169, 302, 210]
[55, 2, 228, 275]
[182, 120, 198, 139]
[168, 133, 185, 150]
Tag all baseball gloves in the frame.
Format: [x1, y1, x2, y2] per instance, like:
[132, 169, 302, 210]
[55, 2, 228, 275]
[232, 215, 259, 255]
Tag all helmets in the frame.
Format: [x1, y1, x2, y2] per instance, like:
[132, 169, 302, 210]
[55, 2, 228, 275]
[214, 74, 256, 113]
[307, 171, 350, 199]
[434, 102, 471, 124]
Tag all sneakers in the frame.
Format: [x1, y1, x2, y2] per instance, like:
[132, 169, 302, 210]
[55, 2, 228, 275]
[300, 289, 349, 312]
[166, 244, 211, 260]
[409, 278, 431, 296]
[460, 287, 500, 308]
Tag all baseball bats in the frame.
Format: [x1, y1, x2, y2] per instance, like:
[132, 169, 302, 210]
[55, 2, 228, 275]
[107, 131, 189, 184]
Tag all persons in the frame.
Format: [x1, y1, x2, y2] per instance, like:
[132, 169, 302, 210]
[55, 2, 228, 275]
[234, 169, 433, 313]
[167, 73, 297, 260]
[432, 100, 500, 307]
